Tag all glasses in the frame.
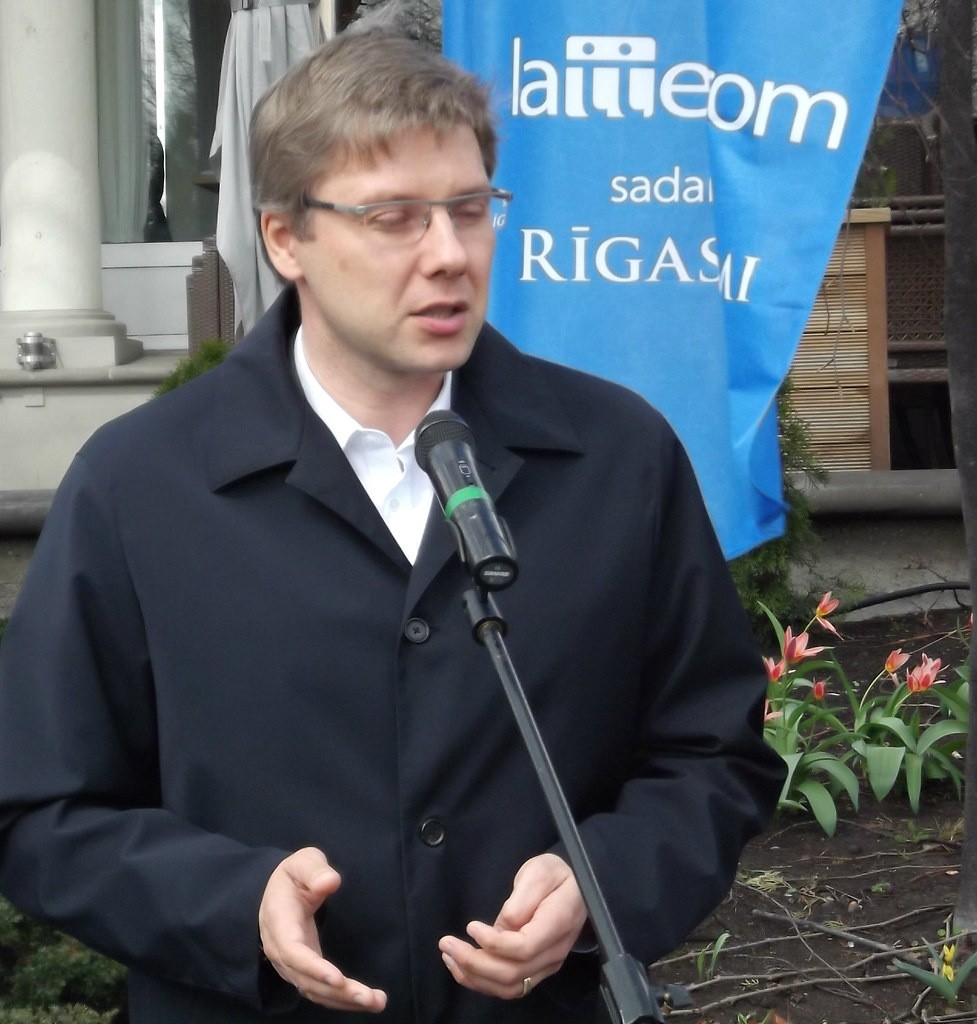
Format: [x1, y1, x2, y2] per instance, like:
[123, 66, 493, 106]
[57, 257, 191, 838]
[300, 188, 514, 246]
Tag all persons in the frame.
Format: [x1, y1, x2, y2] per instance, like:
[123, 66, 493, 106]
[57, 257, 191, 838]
[0, 21, 789, 1024]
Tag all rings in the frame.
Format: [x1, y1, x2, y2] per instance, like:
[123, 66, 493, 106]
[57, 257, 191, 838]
[515, 976, 531, 999]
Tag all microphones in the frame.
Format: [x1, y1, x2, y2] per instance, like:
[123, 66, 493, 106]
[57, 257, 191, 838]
[414, 409, 519, 592]
